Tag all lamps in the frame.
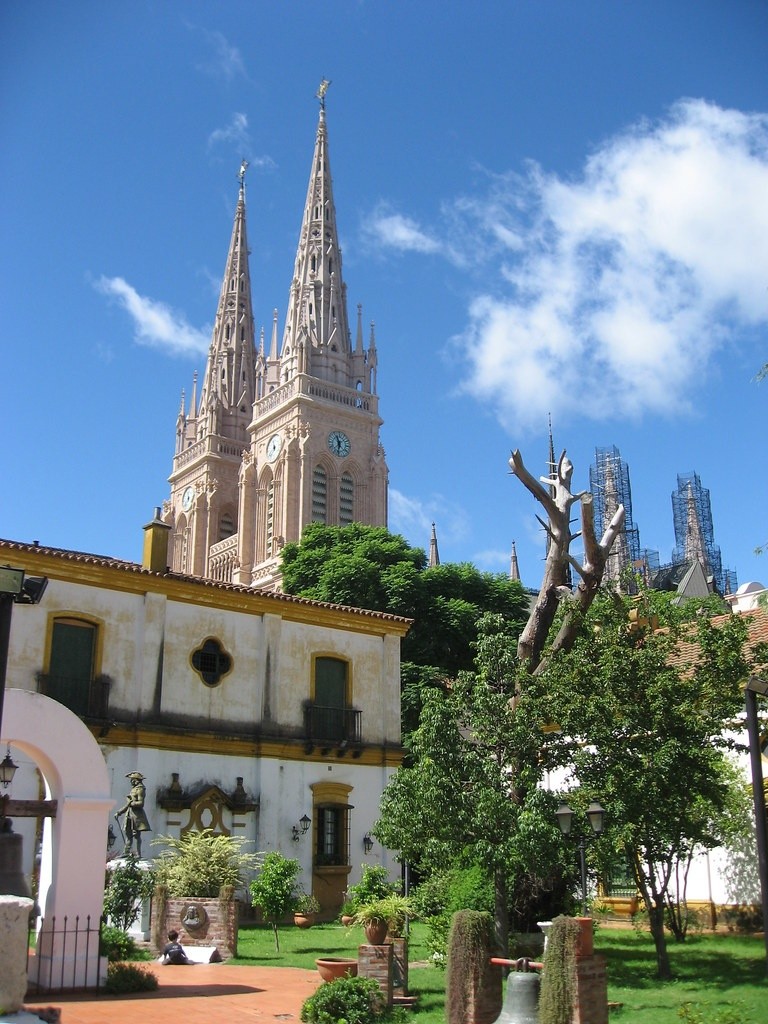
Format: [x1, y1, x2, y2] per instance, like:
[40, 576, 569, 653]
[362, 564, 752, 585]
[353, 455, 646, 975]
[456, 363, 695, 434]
[293, 814, 311, 841]
[364, 834, 374, 855]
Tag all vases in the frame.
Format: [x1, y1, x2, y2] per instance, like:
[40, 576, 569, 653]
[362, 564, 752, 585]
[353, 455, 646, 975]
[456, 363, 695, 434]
[314, 958, 359, 983]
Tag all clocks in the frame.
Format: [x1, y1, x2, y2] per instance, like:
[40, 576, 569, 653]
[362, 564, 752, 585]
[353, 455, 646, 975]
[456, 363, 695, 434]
[266, 432, 283, 463]
[328, 431, 353, 458]
[181, 486, 197, 511]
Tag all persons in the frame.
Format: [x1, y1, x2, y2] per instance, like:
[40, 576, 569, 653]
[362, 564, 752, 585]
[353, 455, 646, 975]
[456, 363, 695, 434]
[161, 930, 194, 966]
[182, 906, 202, 930]
[113, 771, 152, 859]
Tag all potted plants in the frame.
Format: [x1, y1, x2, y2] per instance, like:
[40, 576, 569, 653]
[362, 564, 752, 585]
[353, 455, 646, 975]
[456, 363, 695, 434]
[338, 900, 357, 927]
[351, 891, 420, 946]
[291, 892, 321, 929]
